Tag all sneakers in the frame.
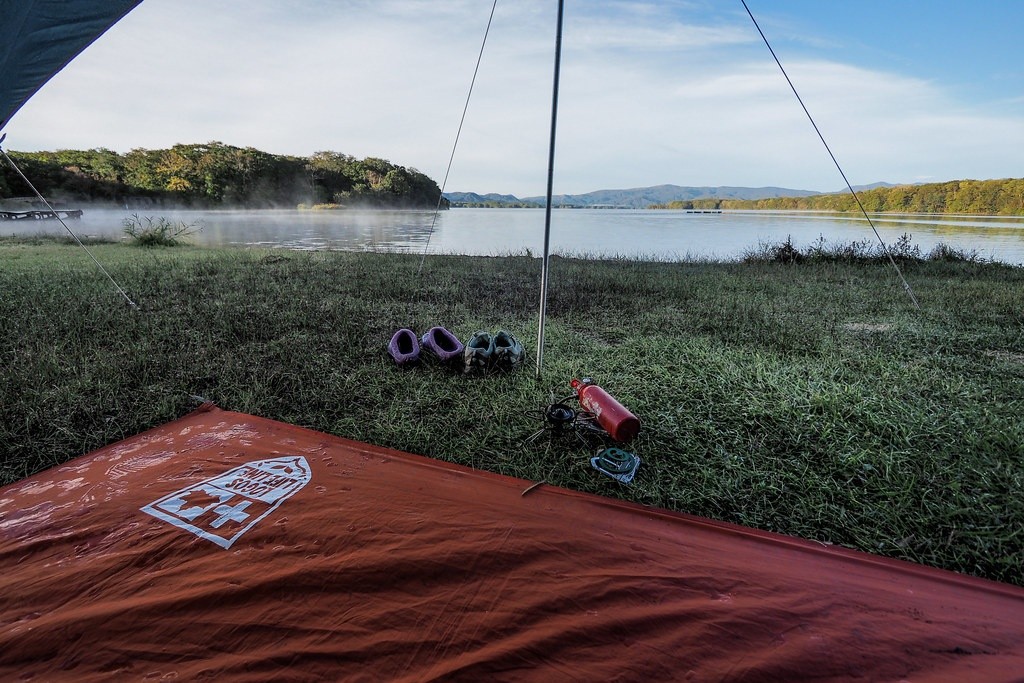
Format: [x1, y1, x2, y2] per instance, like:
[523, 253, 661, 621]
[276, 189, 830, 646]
[492, 330, 522, 369]
[463, 330, 493, 374]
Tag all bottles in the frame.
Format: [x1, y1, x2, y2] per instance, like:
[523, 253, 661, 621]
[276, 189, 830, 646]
[570, 379, 642, 444]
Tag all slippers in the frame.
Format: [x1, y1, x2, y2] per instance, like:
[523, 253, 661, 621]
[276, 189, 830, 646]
[387, 329, 420, 365]
[422, 327, 463, 360]
